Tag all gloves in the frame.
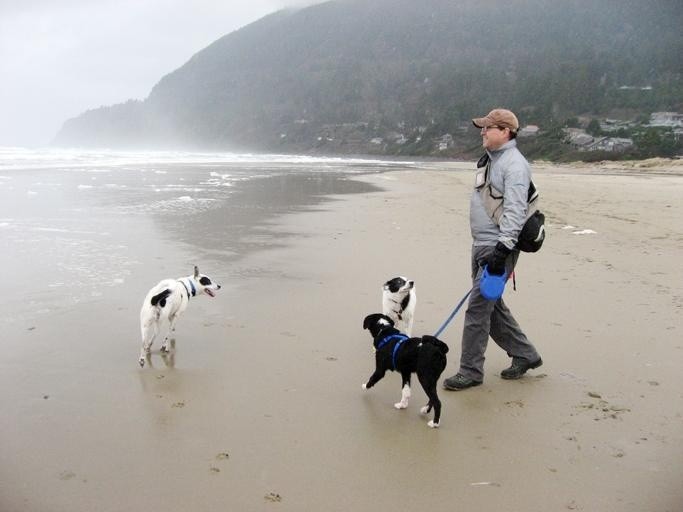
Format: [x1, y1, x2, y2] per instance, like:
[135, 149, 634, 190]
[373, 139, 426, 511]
[479, 241, 510, 276]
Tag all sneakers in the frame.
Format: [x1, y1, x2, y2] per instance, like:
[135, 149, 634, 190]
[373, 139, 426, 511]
[442, 373, 482, 391]
[500, 357, 542, 379]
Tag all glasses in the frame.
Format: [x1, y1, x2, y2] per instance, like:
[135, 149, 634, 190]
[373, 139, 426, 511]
[481, 126, 498, 131]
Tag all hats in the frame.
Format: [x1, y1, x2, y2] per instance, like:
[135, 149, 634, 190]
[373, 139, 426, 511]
[471, 108, 518, 133]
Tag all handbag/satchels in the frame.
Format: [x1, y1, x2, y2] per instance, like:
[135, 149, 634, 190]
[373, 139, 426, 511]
[515, 208, 545, 253]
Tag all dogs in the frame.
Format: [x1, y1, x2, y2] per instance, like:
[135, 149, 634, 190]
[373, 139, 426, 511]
[137, 265, 221, 368]
[381, 276, 416, 339]
[361, 313, 449, 428]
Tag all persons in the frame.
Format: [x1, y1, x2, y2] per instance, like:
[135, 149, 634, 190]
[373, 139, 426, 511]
[443, 109, 546, 390]
